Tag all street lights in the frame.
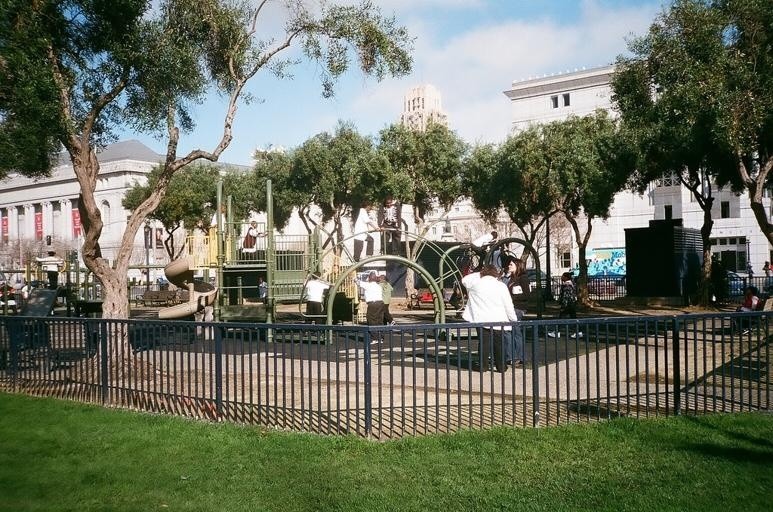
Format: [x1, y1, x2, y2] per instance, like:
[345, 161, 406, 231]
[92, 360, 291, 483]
[744, 239, 754, 285]
[144, 224, 152, 291]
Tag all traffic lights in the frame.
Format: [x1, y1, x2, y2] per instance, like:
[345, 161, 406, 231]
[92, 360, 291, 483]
[47, 235, 51, 245]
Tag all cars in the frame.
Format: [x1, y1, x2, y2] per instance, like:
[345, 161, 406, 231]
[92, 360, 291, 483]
[527, 269, 554, 298]
[573, 275, 627, 294]
[763, 276, 773, 296]
[727, 270, 760, 296]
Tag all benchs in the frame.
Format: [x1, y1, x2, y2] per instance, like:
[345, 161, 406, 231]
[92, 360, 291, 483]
[135, 290, 175, 307]
[412, 288, 456, 308]
[735, 296, 767, 333]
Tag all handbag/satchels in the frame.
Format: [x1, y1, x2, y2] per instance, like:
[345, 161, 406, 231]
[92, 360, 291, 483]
[511, 284, 532, 295]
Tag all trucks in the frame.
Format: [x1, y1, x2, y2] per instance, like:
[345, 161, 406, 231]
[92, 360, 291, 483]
[569, 249, 627, 290]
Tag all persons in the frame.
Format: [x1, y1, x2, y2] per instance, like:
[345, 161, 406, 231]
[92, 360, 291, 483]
[21, 283, 33, 299]
[356, 272, 384, 345]
[377, 193, 404, 257]
[481, 231, 502, 272]
[352, 195, 380, 265]
[505, 256, 530, 366]
[735, 285, 761, 334]
[549, 272, 584, 339]
[377, 274, 395, 326]
[744, 261, 754, 277]
[461, 263, 516, 373]
[35, 250, 63, 289]
[239, 220, 266, 253]
[763, 261, 773, 278]
[328, 264, 346, 285]
[258, 277, 267, 302]
[303, 271, 328, 324]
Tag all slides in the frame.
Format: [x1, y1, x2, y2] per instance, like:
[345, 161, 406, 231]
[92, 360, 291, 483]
[158, 256, 218, 320]
[19, 286, 61, 317]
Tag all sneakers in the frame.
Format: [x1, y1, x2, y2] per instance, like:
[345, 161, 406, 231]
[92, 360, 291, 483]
[571, 332, 584, 339]
[369, 337, 384, 345]
[547, 331, 561, 338]
[388, 321, 397, 325]
